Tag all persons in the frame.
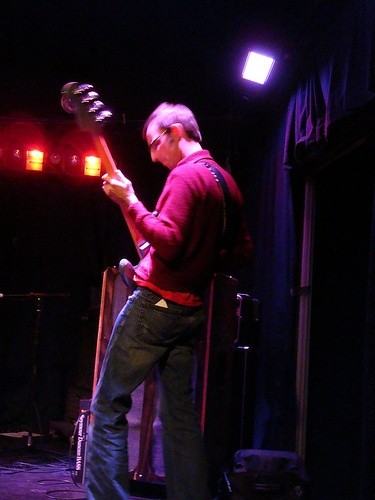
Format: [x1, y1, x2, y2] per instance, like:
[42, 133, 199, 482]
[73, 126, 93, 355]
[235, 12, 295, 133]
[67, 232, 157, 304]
[81, 99, 245, 500]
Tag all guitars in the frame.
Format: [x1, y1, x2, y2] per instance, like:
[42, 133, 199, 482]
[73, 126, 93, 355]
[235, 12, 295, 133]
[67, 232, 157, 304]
[60, 81, 153, 294]
